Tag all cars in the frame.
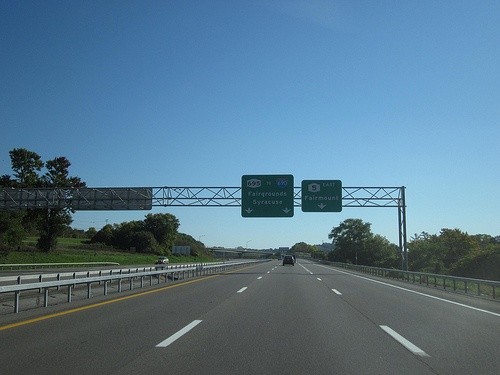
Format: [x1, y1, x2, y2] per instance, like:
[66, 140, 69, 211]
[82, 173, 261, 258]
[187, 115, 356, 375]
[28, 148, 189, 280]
[155, 257, 169, 264]
[283, 255, 294, 266]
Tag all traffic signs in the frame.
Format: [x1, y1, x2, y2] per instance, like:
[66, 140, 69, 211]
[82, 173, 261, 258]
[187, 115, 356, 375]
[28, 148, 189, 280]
[242, 174, 294, 217]
[301, 179, 342, 212]
